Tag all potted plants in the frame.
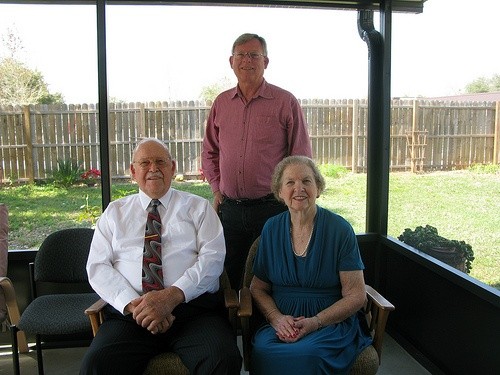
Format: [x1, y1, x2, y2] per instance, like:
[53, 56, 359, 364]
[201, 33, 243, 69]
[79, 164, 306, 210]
[397, 225, 475, 275]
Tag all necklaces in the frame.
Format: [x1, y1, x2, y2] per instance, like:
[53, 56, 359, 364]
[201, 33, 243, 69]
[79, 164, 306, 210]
[290, 227, 310, 257]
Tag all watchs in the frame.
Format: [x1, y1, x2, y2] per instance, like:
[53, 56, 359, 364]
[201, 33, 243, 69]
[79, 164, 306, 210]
[315, 314, 321, 330]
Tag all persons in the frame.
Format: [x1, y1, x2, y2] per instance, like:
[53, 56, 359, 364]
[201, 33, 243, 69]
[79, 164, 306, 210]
[249, 155, 374, 374]
[201, 33, 312, 290]
[78, 137, 243, 375]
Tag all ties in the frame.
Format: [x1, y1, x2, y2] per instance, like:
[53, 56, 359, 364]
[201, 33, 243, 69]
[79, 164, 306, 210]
[142, 199, 164, 293]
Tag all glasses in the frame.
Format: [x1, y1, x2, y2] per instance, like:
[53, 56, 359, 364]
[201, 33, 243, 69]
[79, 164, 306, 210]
[135, 158, 172, 169]
[232, 51, 264, 60]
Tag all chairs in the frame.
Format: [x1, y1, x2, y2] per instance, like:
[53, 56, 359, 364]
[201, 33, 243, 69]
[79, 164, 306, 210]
[0, 202, 29, 353]
[10, 228, 100, 375]
[84, 266, 239, 375]
[236, 235, 395, 375]
